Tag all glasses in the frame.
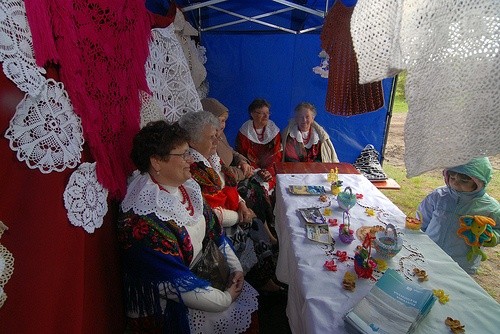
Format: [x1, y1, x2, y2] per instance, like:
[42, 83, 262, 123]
[164, 149, 193, 161]
[253, 110, 271, 115]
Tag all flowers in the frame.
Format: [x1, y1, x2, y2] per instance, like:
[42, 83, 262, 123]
[324, 207, 388, 288]
[414, 268, 428, 282]
[445, 317, 465, 334]
[356, 193, 363, 199]
[320, 194, 327, 201]
[433, 289, 449, 304]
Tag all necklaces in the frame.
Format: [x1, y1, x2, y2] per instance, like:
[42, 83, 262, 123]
[150, 175, 194, 216]
[298, 127, 311, 144]
[254, 120, 266, 133]
[254, 125, 267, 141]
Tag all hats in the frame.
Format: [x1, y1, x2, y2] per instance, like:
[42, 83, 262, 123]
[200, 98, 229, 117]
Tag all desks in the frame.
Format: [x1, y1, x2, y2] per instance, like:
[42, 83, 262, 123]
[274, 163, 500, 334]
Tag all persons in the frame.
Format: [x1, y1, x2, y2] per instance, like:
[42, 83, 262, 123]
[182, 98, 289, 294]
[418, 157, 500, 272]
[281, 102, 341, 163]
[110, 119, 271, 334]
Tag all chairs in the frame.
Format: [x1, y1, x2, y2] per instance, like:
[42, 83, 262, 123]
[113, 202, 178, 334]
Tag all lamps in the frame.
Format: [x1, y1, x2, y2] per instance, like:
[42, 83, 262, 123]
[145, 0, 199, 31]
